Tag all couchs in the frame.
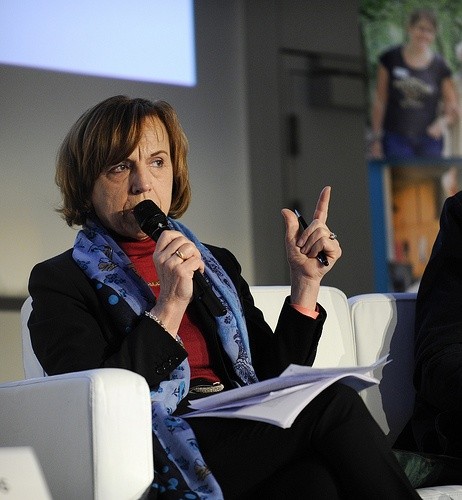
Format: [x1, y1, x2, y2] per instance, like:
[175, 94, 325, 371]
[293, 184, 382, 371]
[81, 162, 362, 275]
[1, 285, 461, 500]
[347, 293, 418, 438]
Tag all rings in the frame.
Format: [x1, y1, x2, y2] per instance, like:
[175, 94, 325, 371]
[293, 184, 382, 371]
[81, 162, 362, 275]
[175, 251, 185, 260]
[329, 232, 337, 240]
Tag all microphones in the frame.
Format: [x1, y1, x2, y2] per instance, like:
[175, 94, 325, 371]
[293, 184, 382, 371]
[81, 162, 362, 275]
[135, 199, 228, 317]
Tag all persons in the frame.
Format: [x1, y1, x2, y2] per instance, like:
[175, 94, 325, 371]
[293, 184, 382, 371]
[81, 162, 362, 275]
[28, 94, 427, 500]
[366, 8, 459, 158]
[412, 191, 462, 487]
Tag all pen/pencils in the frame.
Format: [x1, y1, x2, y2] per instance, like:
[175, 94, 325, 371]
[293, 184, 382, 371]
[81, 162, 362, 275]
[295, 210, 330, 267]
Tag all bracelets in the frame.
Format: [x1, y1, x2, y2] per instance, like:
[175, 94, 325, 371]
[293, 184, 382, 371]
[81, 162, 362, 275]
[143, 308, 179, 342]
